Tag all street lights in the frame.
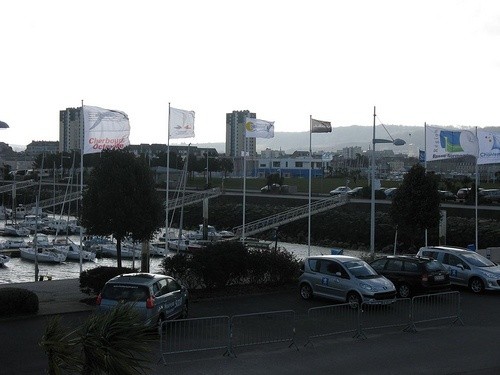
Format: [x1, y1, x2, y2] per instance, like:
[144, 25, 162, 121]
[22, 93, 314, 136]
[370, 105, 406, 254]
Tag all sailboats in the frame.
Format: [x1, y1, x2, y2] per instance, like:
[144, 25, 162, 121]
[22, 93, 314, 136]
[0, 148, 237, 265]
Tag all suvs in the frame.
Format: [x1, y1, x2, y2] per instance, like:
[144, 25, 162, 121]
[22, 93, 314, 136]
[416, 246, 500, 294]
[367, 256, 451, 299]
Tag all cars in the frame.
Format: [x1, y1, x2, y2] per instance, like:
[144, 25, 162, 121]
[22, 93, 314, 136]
[347, 186, 364, 197]
[480, 187, 500, 202]
[439, 190, 459, 202]
[261, 183, 289, 194]
[299, 256, 397, 311]
[94, 272, 189, 337]
[457, 187, 483, 200]
[378, 186, 398, 200]
[330, 186, 351, 196]
